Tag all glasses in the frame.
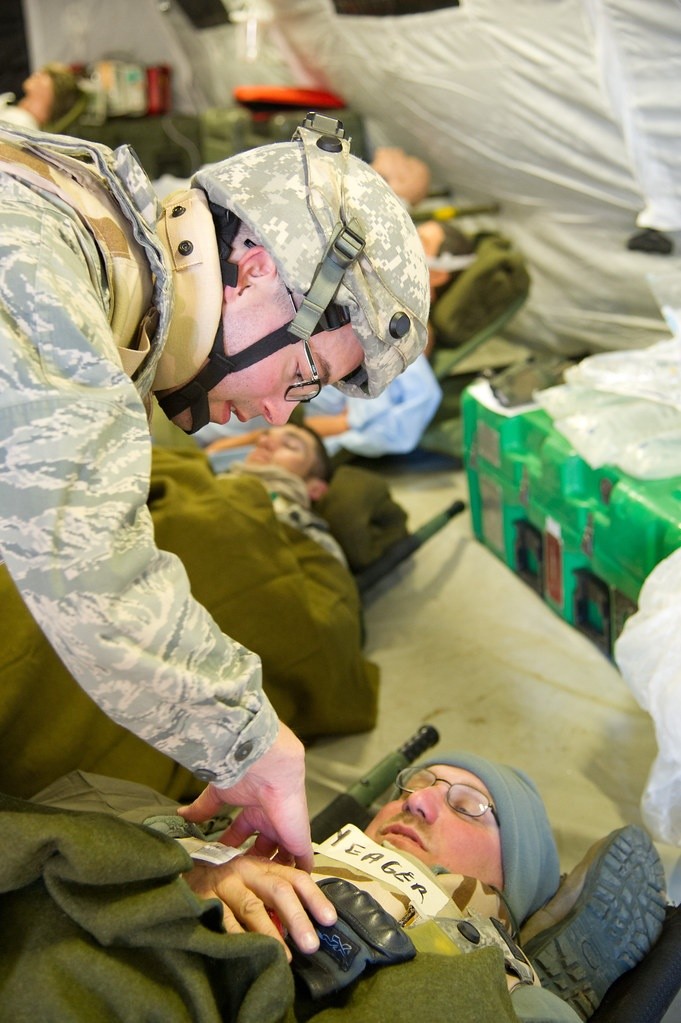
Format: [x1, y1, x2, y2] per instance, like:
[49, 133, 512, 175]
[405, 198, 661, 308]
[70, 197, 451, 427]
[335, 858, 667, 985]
[396, 766, 499, 828]
[243, 237, 321, 402]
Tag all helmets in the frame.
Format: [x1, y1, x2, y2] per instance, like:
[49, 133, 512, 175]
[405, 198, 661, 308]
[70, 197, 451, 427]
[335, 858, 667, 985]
[188, 140, 430, 398]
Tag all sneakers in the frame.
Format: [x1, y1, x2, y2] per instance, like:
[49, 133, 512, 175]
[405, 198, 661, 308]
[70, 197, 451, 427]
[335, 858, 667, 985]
[519, 822, 667, 1019]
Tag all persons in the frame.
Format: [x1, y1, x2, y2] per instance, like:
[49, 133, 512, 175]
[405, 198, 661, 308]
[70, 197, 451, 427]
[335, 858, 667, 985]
[220, 422, 347, 568]
[191, 219, 469, 462]
[0, 68, 80, 131]
[0, 754, 582, 1023]
[371, 142, 428, 204]
[417, 220, 466, 289]
[0, 111, 432, 871]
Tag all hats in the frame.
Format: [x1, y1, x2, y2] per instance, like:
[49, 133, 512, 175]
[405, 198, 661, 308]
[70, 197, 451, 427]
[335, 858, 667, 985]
[390, 756, 560, 918]
